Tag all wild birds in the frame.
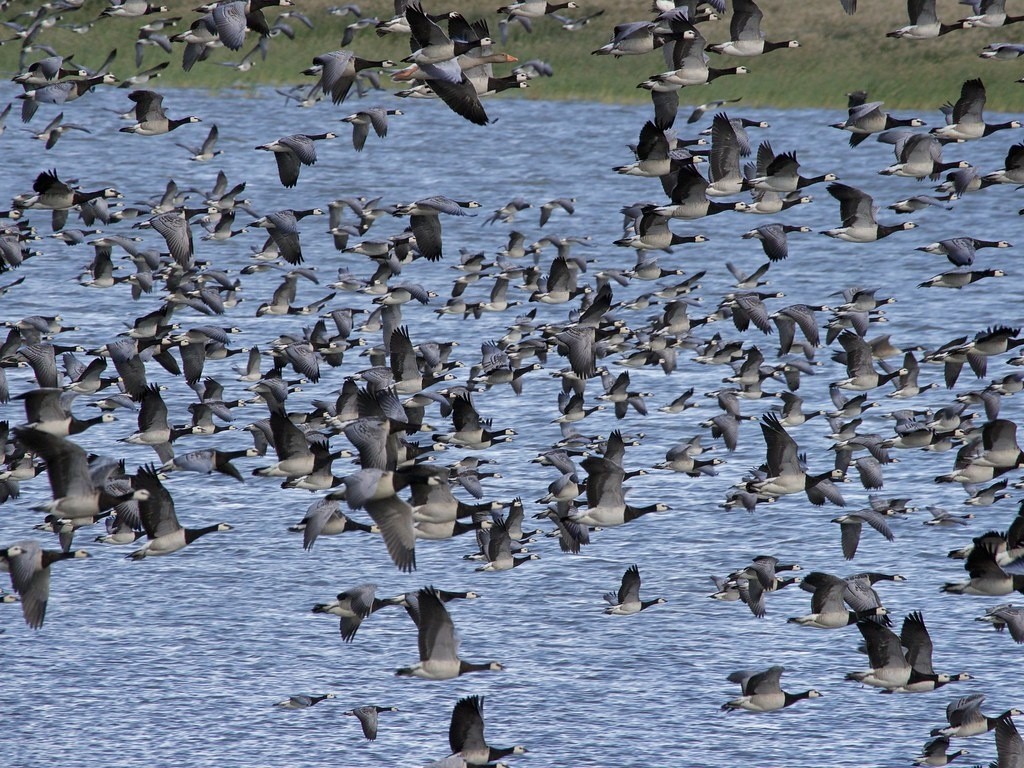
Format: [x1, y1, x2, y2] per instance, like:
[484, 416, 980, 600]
[930, 694, 1024, 737]
[601, 565, 668, 614]
[706, 556, 805, 619]
[785, 571, 908, 628]
[720, 666, 824, 713]
[844, 617, 957, 690]
[312, 583, 482, 644]
[939, 499, 1024, 645]
[2, 0, 1024, 574]
[273, 694, 338, 709]
[423, 695, 530, 768]
[394, 585, 507, 681]
[877, 611, 975, 694]
[343, 706, 400, 742]
[0, 539, 93, 630]
[908, 736, 970, 766]
[474, 518, 541, 572]
[987, 714, 1024, 768]
